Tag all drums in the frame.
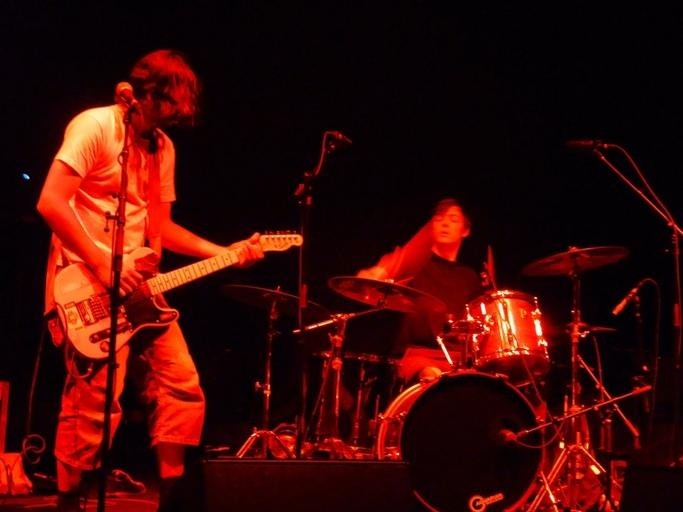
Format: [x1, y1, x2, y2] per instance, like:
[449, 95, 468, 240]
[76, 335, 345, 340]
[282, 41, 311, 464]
[462, 291, 550, 389]
[380, 367, 544, 512]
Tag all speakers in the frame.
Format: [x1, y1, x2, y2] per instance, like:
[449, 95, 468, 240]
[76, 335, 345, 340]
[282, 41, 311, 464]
[620, 463, 683, 512]
[156, 459, 411, 512]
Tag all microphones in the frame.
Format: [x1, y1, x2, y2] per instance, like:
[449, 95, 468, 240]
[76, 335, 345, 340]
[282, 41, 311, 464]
[567, 139, 609, 150]
[330, 130, 353, 145]
[115, 81, 141, 114]
[611, 281, 644, 316]
[498, 428, 517, 447]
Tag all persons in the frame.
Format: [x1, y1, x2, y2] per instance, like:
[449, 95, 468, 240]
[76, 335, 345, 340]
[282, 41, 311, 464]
[32, 49, 265, 511]
[360, 195, 492, 354]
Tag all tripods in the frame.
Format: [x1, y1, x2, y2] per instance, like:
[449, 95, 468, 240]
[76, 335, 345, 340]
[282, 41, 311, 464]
[292, 304, 378, 462]
[232, 300, 296, 459]
[523, 282, 625, 512]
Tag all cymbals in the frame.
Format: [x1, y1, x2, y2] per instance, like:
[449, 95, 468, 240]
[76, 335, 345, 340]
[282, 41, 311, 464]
[524, 245, 630, 276]
[557, 325, 615, 335]
[227, 282, 323, 311]
[328, 275, 430, 315]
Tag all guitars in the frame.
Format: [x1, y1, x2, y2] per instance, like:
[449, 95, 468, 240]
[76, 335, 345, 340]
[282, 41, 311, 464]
[52, 229, 303, 360]
